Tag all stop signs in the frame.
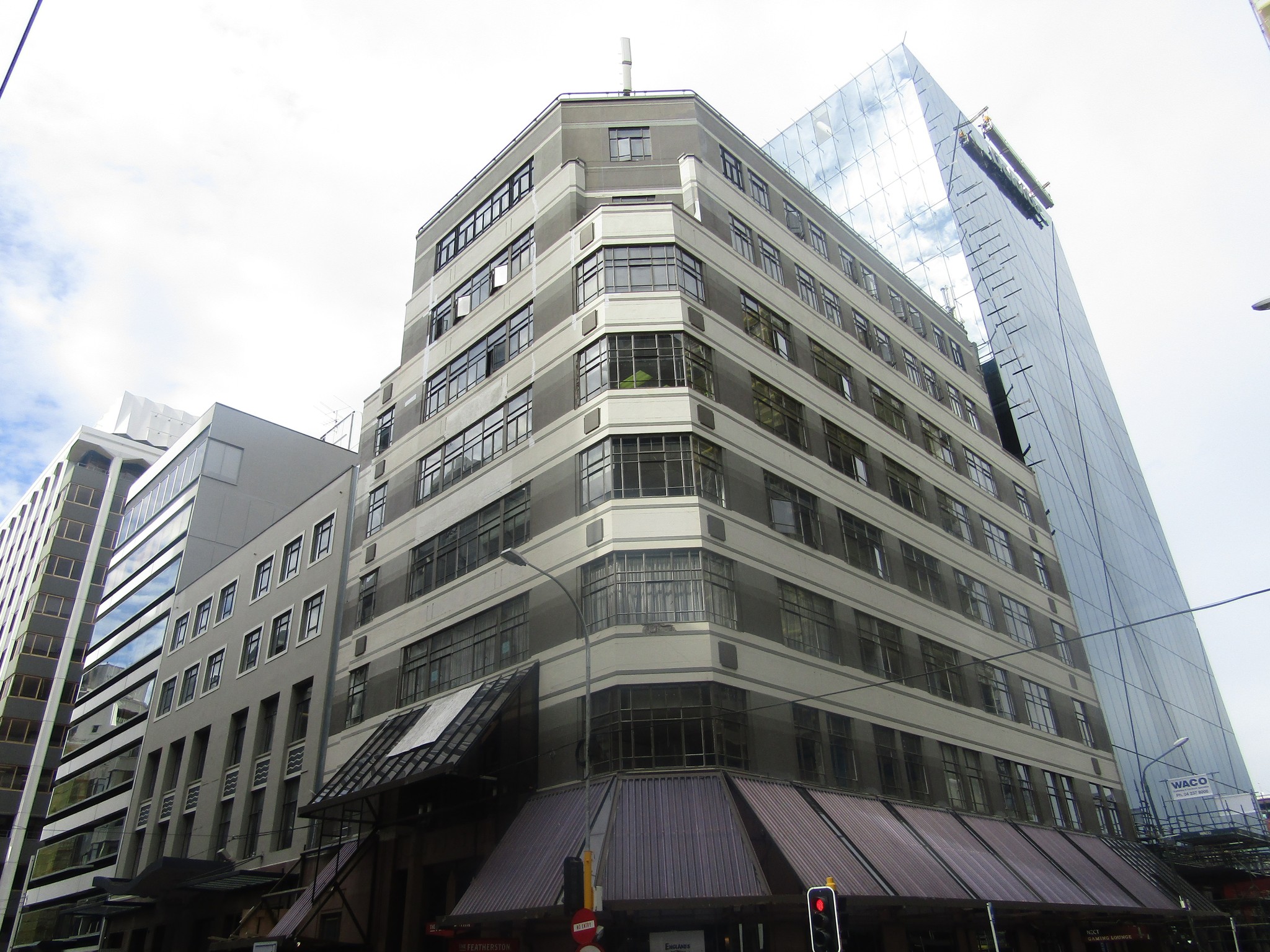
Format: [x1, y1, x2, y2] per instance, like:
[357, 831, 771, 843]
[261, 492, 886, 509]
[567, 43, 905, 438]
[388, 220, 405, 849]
[571, 908, 597, 945]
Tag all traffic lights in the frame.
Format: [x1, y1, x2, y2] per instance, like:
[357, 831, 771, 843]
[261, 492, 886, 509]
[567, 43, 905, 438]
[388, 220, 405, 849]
[809, 888, 843, 952]
[564, 857, 585, 913]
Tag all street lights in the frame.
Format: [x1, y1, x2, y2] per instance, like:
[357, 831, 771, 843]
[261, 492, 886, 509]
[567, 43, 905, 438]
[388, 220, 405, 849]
[1142, 737, 1204, 952]
[499, 547, 594, 912]
